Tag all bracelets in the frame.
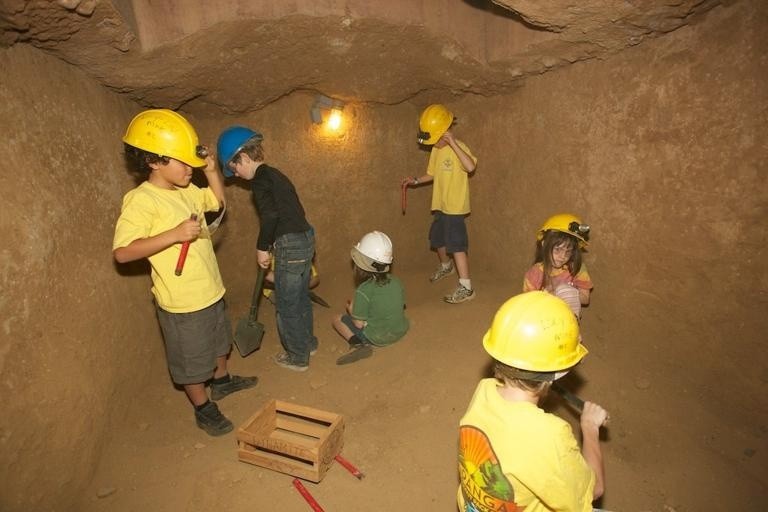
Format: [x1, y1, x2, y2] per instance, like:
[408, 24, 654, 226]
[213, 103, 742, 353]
[413, 177, 418, 186]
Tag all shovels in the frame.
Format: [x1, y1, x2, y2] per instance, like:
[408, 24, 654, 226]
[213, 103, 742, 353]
[233, 267, 264, 358]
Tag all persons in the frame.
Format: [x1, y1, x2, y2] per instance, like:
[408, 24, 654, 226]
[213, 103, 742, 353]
[111, 108, 257, 438]
[326, 228, 410, 366]
[215, 125, 319, 373]
[261, 260, 320, 302]
[450, 291, 607, 511]
[399, 104, 478, 304]
[523, 213, 593, 329]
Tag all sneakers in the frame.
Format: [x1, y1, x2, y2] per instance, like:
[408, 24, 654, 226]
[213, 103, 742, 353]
[209, 374, 258, 401]
[335, 344, 374, 366]
[442, 281, 476, 305]
[194, 401, 234, 437]
[428, 258, 456, 283]
[273, 335, 318, 372]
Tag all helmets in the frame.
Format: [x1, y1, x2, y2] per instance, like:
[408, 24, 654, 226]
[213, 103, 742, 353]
[416, 103, 454, 146]
[536, 212, 592, 249]
[217, 126, 257, 178]
[121, 108, 208, 169]
[482, 289, 590, 374]
[350, 230, 393, 274]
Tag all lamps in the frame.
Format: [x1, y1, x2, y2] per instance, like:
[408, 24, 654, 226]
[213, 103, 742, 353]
[328, 98, 346, 131]
